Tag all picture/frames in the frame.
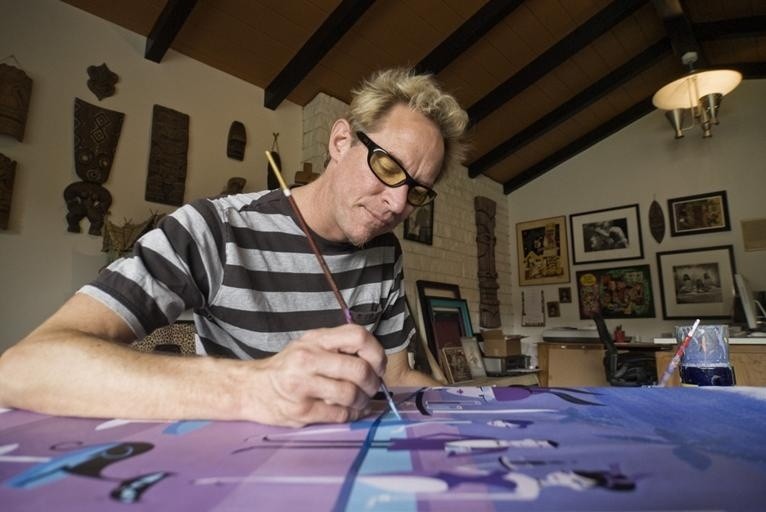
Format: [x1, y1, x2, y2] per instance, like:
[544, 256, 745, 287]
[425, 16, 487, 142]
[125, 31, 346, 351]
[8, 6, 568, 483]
[416, 280, 488, 384]
[512, 190, 741, 323]
[403, 189, 434, 247]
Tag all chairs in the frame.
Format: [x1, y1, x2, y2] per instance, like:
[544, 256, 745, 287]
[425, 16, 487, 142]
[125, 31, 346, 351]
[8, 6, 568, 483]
[590, 312, 654, 386]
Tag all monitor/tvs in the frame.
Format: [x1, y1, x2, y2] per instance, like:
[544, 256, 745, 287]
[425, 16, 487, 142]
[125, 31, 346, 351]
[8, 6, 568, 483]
[734, 273, 766, 336]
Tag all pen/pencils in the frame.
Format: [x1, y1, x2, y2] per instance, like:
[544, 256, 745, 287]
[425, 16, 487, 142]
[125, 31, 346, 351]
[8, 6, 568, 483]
[658, 319, 700, 387]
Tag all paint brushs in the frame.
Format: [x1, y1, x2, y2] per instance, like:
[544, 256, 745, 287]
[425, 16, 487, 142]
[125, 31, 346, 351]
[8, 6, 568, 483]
[266, 152, 403, 421]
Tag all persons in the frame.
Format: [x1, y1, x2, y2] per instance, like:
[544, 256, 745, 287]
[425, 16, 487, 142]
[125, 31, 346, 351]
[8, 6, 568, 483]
[695, 278, 709, 293]
[228, 432, 560, 461]
[678, 273, 693, 294]
[367, 384, 552, 406]
[283, 415, 536, 436]
[184, 460, 639, 510]
[702, 272, 721, 290]
[0, 67, 472, 426]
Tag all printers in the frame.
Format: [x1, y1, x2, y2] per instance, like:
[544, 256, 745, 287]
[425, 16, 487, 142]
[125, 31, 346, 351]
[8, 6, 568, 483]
[481, 354, 541, 375]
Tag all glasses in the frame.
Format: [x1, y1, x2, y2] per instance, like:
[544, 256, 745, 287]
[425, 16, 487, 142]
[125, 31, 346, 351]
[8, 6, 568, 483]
[357, 129, 439, 207]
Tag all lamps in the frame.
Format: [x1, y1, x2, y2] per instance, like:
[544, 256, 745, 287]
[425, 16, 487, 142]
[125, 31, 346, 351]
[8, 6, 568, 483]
[649, 49, 745, 140]
